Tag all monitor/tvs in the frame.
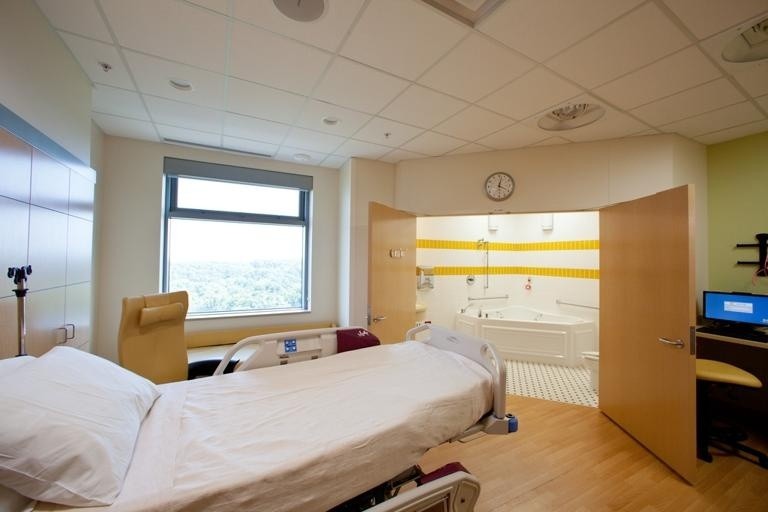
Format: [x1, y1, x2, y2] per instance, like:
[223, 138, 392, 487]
[701, 289, 768, 329]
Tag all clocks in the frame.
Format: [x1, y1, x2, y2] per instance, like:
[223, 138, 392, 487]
[484, 172, 515, 201]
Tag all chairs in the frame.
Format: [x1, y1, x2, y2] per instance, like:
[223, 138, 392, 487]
[119, 291, 189, 384]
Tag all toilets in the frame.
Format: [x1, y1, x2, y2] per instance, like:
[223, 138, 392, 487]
[582, 351, 599, 391]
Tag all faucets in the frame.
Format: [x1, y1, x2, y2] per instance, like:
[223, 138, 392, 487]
[461, 303, 474, 314]
[464, 303, 473, 310]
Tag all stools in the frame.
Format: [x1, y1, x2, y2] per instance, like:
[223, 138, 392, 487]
[696, 359, 764, 462]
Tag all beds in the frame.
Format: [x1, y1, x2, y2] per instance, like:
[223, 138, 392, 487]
[0, 324, 518, 512]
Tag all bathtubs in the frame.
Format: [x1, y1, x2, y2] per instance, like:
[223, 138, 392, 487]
[456, 306, 596, 366]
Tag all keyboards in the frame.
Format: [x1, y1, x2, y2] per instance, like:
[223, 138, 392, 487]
[696, 324, 768, 345]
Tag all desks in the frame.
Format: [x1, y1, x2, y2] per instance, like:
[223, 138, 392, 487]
[187, 344, 258, 366]
[696, 321, 768, 361]
